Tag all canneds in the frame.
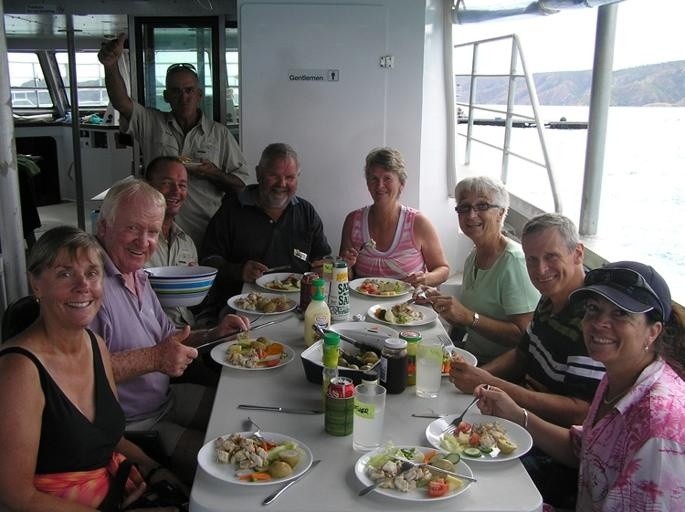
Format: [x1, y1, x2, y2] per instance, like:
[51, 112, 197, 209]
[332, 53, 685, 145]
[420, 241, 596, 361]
[380, 331, 422, 395]
[327, 376, 354, 401]
[300, 271, 319, 313]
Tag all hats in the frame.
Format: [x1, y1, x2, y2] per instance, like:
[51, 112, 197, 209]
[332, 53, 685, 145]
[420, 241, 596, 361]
[569, 261, 671, 321]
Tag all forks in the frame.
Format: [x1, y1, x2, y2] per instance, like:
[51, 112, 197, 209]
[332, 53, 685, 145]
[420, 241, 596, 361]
[389, 455, 478, 483]
[357, 460, 418, 498]
[437, 334, 453, 346]
[444, 384, 490, 433]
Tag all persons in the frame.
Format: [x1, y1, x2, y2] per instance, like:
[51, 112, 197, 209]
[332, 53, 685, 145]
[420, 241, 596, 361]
[338, 146, 450, 290]
[413, 175, 542, 367]
[82, 177, 217, 478]
[97, 30, 249, 259]
[202, 142, 335, 312]
[0, 224, 191, 511]
[448, 213, 608, 512]
[473, 261, 685, 511]
[138, 155, 237, 387]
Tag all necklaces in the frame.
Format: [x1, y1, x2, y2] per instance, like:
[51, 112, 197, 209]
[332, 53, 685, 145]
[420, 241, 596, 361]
[602, 380, 634, 405]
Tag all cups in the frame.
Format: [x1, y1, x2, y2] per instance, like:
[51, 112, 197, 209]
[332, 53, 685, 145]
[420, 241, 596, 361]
[351, 385, 386, 455]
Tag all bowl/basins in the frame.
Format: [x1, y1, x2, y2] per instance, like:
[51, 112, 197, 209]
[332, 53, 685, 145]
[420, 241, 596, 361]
[144, 264, 218, 309]
[300, 337, 385, 387]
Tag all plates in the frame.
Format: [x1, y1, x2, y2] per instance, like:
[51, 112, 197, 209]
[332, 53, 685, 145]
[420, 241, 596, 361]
[179, 158, 207, 171]
[425, 414, 530, 463]
[255, 272, 304, 292]
[348, 277, 414, 297]
[209, 337, 296, 370]
[226, 292, 298, 316]
[197, 431, 315, 486]
[354, 447, 472, 502]
[442, 346, 478, 375]
[366, 302, 439, 327]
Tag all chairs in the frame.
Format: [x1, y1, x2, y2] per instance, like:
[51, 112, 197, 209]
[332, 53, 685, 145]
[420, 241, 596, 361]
[0, 296, 172, 466]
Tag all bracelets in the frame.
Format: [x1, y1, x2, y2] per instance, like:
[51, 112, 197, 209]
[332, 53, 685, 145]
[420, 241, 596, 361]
[144, 464, 165, 483]
[522, 407, 529, 430]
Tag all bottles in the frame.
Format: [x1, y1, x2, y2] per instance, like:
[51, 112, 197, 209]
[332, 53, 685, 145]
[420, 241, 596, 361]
[303, 254, 351, 344]
[321, 333, 340, 412]
[361, 330, 442, 400]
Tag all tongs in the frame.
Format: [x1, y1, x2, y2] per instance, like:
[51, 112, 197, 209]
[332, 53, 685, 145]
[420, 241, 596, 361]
[312, 323, 380, 366]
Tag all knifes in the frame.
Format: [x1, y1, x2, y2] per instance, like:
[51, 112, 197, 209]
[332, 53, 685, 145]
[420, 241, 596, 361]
[265, 264, 290, 272]
[236, 404, 321, 415]
[263, 458, 322, 504]
[385, 261, 408, 276]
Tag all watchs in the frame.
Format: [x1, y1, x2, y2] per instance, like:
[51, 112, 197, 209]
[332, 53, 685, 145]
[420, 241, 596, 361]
[471, 312, 480, 330]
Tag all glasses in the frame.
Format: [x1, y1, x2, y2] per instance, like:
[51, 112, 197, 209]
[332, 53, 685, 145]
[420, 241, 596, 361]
[455, 203, 500, 213]
[584, 269, 665, 327]
[167, 63, 196, 72]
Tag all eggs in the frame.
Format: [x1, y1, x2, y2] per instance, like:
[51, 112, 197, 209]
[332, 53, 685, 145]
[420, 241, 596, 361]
[338, 349, 378, 373]
[497, 438, 518, 454]
[433, 459, 453, 472]
[269, 447, 300, 479]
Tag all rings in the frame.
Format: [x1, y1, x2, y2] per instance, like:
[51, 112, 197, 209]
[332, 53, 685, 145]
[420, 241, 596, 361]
[452, 376, 455, 383]
[442, 306, 445, 312]
